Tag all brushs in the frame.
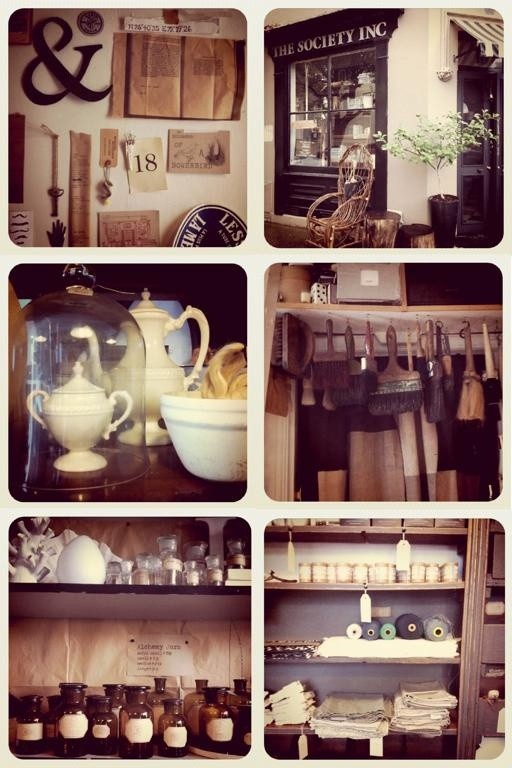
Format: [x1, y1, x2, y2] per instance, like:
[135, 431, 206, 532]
[271, 313, 501, 430]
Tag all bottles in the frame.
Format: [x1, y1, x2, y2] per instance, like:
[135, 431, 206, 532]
[8, 676, 252, 758]
[298, 560, 460, 586]
[107, 530, 249, 587]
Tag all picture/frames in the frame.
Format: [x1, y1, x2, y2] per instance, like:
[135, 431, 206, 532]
[9, 9, 33, 46]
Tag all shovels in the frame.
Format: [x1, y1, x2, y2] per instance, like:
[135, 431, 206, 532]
[265, 364, 289, 417]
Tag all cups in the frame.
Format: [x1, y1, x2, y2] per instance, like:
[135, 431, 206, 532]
[352, 124, 365, 139]
[361, 95, 374, 109]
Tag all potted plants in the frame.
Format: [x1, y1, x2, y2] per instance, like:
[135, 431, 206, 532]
[372, 109, 502, 247]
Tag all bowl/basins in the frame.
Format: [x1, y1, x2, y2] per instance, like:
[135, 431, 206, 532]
[158, 387, 248, 483]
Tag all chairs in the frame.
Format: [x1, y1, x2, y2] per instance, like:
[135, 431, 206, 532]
[304, 143, 376, 248]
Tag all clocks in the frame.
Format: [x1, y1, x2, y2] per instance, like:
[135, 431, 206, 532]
[77, 10, 104, 36]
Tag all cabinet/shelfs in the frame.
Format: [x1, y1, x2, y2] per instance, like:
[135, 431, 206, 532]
[264, 518, 479, 760]
[467, 519, 505, 757]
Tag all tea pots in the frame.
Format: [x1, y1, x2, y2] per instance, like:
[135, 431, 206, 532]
[84, 287, 211, 447]
